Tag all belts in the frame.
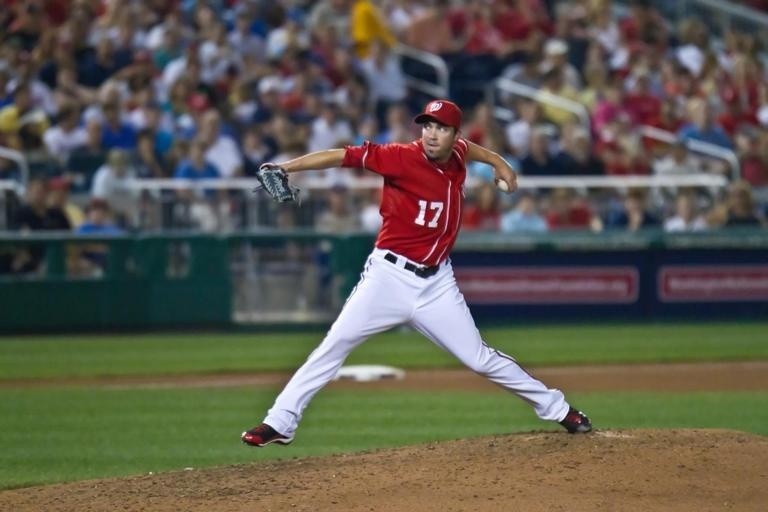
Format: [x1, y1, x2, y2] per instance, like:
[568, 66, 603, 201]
[385, 254, 439, 278]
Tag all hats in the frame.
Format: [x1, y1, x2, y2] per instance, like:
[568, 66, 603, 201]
[414, 100, 462, 130]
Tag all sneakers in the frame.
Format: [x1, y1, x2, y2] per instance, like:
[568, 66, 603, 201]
[558, 406, 591, 434]
[241, 424, 294, 447]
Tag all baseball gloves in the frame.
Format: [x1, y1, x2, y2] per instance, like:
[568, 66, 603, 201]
[255, 165, 301, 203]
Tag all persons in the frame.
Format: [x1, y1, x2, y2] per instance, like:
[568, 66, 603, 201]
[0, 0, 768, 279]
[239, 100, 594, 449]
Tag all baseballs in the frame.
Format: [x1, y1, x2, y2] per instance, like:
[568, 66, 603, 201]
[497, 178, 508, 192]
